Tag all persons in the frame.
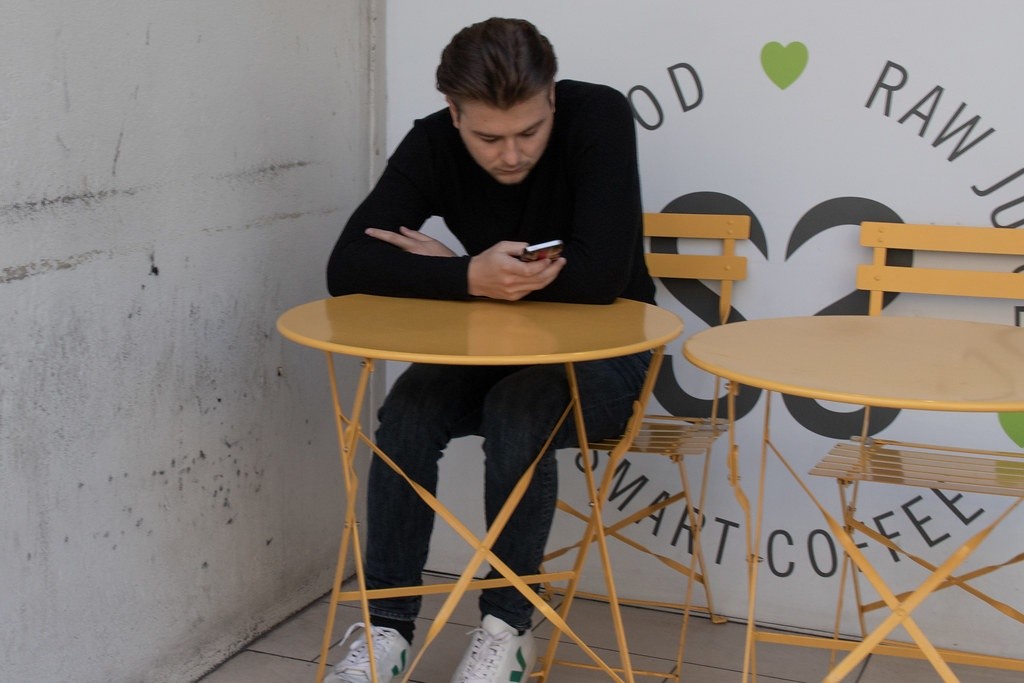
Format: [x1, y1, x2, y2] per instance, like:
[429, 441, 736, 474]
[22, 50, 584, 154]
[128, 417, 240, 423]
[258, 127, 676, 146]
[324, 18, 656, 683]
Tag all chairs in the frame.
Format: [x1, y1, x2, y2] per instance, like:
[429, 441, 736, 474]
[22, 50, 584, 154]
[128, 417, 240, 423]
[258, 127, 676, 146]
[536, 213, 751, 683]
[807, 219, 1024, 674]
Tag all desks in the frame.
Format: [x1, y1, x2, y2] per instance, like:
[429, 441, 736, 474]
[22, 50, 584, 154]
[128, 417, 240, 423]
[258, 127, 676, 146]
[682, 313, 1024, 683]
[276, 293, 686, 683]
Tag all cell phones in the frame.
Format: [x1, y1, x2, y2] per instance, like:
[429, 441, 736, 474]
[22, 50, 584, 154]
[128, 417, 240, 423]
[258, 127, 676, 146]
[522, 240, 563, 264]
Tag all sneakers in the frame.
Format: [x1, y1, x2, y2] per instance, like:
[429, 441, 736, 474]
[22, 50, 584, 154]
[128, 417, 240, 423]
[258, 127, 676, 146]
[452, 613, 536, 683]
[324, 621, 410, 682]
